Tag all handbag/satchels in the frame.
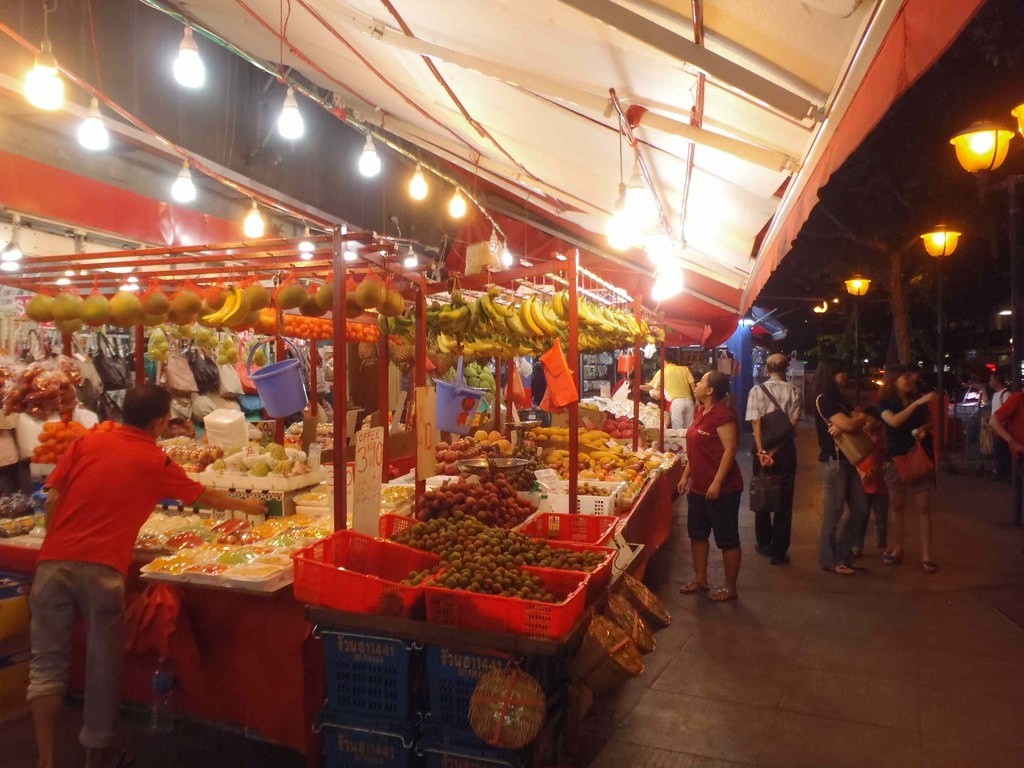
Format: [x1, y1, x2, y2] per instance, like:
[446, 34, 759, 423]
[505, 362, 525, 403]
[891, 439, 934, 485]
[711, 350, 740, 376]
[751, 408, 797, 455]
[22, 328, 327, 421]
[649, 377, 661, 400]
[758, 384, 796, 449]
[816, 393, 876, 466]
[749, 463, 782, 513]
[613, 382, 631, 402]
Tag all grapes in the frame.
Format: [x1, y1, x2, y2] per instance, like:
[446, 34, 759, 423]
[505, 441, 544, 491]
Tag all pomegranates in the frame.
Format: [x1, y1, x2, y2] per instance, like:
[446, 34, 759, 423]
[549, 458, 649, 479]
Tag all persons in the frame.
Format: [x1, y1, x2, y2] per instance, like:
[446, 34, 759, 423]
[744, 354, 803, 564]
[854, 404, 891, 558]
[639, 351, 696, 431]
[872, 363, 942, 573]
[989, 390, 1024, 527]
[25, 383, 269, 767]
[812, 356, 871, 575]
[677, 371, 743, 601]
[987, 373, 1013, 484]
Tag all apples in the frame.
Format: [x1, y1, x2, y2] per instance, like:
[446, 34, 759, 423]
[434, 437, 496, 476]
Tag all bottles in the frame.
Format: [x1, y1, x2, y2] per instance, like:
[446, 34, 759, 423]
[150, 657, 175, 735]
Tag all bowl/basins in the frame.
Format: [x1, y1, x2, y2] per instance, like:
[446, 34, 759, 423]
[137, 480, 416, 592]
[456, 458, 529, 474]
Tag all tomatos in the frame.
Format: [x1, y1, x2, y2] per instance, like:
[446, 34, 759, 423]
[155, 443, 221, 472]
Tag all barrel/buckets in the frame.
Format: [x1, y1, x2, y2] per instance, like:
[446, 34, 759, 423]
[618, 349, 636, 374]
[432, 355, 485, 435]
[246, 337, 309, 418]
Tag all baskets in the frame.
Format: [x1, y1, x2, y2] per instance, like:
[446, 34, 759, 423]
[289, 469, 627, 641]
[572, 574, 671, 698]
[312, 625, 568, 768]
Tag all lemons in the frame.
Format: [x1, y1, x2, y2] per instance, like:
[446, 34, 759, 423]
[147, 325, 266, 365]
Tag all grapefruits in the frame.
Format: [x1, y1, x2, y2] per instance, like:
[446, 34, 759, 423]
[24, 272, 405, 331]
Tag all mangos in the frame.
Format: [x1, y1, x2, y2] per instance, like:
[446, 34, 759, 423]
[577, 469, 644, 499]
[522, 426, 662, 468]
[213, 441, 298, 478]
[449, 362, 496, 403]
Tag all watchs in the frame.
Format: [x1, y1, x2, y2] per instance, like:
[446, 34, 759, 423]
[759, 450, 766, 454]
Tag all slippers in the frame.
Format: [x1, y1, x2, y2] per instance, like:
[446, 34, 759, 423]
[883, 554, 905, 564]
[708, 589, 738, 602]
[112, 749, 135, 768]
[680, 580, 710, 594]
[921, 560, 936, 572]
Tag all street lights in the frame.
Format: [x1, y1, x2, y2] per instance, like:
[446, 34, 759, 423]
[920, 216, 964, 500]
[947, 117, 1018, 475]
[842, 265, 872, 402]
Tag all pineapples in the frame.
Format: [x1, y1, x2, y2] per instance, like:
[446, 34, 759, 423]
[359, 341, 477, 368]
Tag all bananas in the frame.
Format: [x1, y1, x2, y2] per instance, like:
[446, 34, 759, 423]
[378, 287, 666, 359]
[202, 284, 249, 328]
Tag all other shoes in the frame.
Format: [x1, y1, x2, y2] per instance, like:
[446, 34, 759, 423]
[823, 561, 866, 575]
[852, 550, 863, 559]
[755, 544, 790, 565]
[883, 549, 890, 556]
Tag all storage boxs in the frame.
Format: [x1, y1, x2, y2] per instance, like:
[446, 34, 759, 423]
[0, 356, 705, 768]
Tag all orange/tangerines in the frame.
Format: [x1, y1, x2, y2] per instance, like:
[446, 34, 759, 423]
[474, 430, 514, 452]
[32, 422, 88, 466]
[269, 310, 380, 342]
